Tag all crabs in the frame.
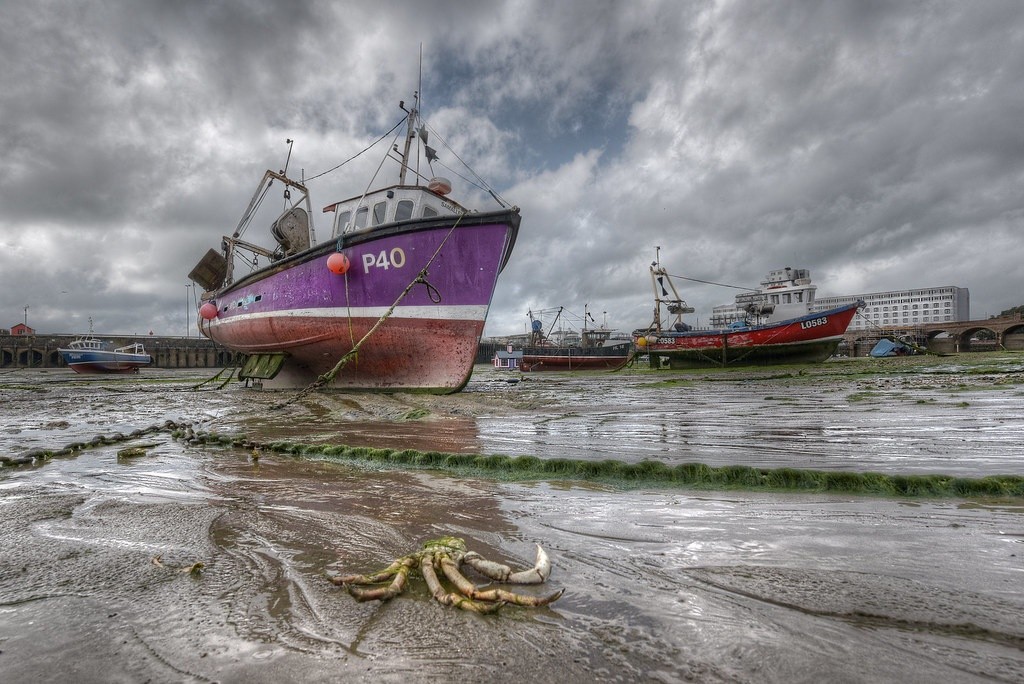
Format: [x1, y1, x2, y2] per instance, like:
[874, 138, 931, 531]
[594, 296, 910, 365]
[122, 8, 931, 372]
[321, 535, 565, 616]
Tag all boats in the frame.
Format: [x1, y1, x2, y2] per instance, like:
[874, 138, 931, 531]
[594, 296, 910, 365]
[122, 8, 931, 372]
[491, 305, 631, 370]
[188, 41, 522, 393]
[57, 317, 151, 375]
[631, 243, 866, 370]
